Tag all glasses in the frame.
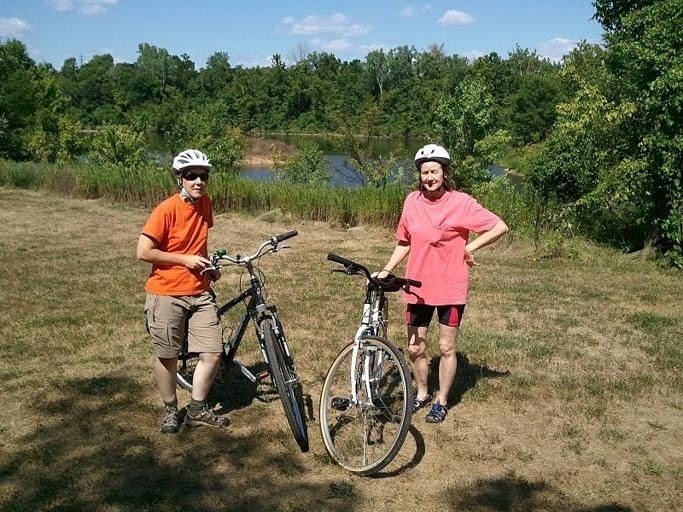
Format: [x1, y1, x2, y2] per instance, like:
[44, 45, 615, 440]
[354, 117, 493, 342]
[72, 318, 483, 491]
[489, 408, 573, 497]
[179, 173, 209, 181]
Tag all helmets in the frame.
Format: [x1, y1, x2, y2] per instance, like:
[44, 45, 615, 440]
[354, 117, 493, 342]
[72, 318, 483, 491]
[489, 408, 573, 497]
[170, 148, 213, 172]
[413, 143, 450, 167]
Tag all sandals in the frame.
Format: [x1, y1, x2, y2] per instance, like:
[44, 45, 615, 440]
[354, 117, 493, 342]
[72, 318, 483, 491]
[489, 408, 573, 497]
[422, 398, 450, 424]
[412, 395, 430, 416]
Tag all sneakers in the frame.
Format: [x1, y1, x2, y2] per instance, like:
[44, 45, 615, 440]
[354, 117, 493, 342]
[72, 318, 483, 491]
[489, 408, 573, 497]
[182, 405, 230, 430]
[160, 411, 179, 432]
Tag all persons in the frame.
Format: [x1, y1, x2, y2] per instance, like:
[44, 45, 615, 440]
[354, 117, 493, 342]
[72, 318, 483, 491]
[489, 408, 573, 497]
[378, 143, 509, 423]
[135, 149, 229, 433]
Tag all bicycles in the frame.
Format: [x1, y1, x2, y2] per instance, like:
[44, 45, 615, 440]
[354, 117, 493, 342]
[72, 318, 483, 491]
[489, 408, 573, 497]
[169, 229, 309, 450]
[318, 250, 423, 477]
